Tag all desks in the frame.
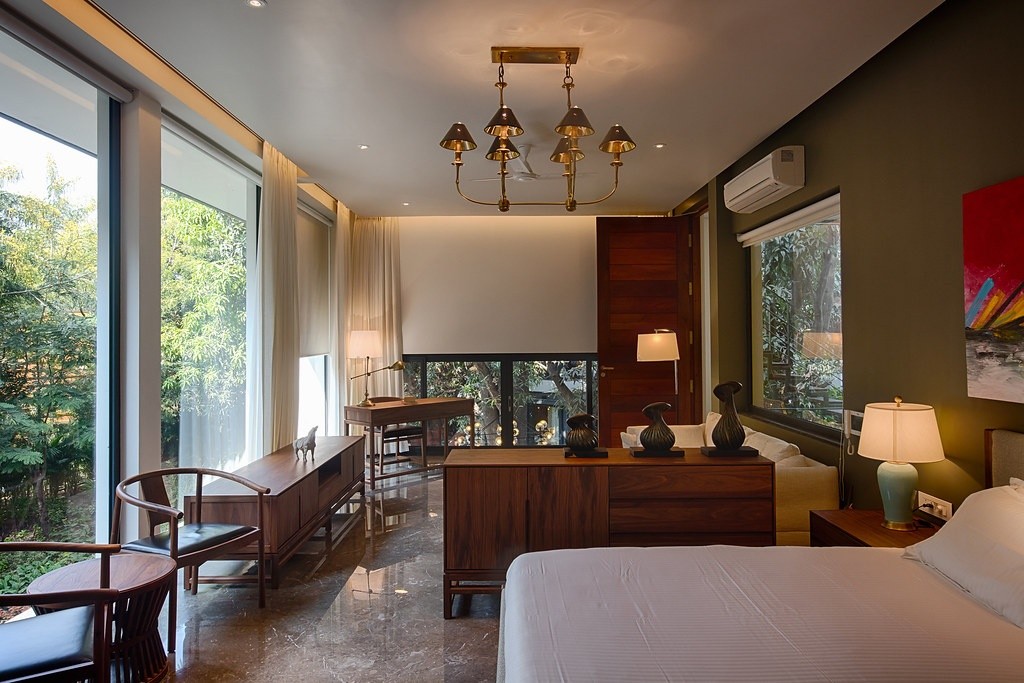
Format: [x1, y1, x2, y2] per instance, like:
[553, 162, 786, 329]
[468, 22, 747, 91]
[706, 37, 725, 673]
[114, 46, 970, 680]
[343, 396, 474, 491]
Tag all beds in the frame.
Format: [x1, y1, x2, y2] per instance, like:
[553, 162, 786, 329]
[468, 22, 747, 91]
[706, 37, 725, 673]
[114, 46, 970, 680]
[494, 429, 1024, 683]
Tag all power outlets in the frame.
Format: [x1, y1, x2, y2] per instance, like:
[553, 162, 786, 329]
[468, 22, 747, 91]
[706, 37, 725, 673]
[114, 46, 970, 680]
[918, 491, 952, 522]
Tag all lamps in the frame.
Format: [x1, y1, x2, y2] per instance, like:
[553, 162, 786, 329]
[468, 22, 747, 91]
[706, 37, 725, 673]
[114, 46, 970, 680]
[636, 328, 680, 425]
[348, 331, 406, 406]
[439, 46, 636, 211]
[857, 396, 946, 530]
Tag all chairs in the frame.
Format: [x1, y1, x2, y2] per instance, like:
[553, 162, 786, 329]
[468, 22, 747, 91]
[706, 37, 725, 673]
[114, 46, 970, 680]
[363, 398, 424, 475]
[110, 467, 272, 653]
[0, 541, 123, 683]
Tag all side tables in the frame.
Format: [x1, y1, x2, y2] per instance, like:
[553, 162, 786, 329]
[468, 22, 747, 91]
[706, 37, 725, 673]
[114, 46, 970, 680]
[26, 554, 177, 683]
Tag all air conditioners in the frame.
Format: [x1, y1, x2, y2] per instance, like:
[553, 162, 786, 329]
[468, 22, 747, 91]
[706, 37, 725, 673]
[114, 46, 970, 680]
[724, 146, 804, 213]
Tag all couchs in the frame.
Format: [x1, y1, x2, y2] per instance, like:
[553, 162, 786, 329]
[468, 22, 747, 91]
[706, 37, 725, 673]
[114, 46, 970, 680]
[621, 412, 840, 546]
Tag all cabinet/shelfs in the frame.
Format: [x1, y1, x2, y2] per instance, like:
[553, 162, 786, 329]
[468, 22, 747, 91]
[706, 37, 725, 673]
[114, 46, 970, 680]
[442, 448, 776, 620]
[183, 437, 365, 590]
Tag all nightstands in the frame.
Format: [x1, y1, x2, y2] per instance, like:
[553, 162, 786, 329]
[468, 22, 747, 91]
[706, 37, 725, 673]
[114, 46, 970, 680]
[809, 509, 941, 548]
